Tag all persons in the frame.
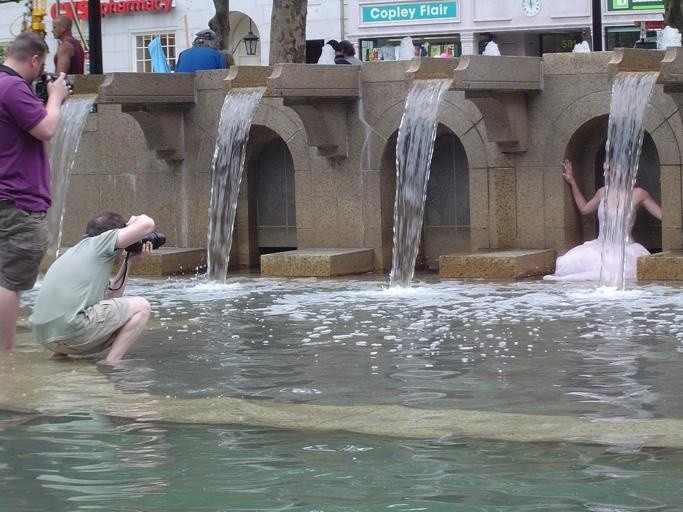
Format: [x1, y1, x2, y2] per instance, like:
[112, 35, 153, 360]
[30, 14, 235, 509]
[340, 40, 361, 63]
[30, 212, 155, 363]
[51, 15, 84, 75]
[543, 154, 662, 282]
[0, 31, 73, 351]
[173, 29, 226, 74]
[326, 39, 352, 65]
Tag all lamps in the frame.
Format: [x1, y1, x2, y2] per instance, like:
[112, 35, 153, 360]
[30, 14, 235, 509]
[242, 17, 259, 55]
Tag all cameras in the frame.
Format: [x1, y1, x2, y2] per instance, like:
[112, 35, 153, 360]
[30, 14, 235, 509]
[124, 224, 165, 252]
[36, 73, 73, 99]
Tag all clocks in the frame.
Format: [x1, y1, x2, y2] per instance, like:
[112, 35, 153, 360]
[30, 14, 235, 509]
[521, 0, 542, 16]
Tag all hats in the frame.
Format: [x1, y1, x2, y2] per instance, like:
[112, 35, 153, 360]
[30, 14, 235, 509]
[196, 30, 216, 39]
